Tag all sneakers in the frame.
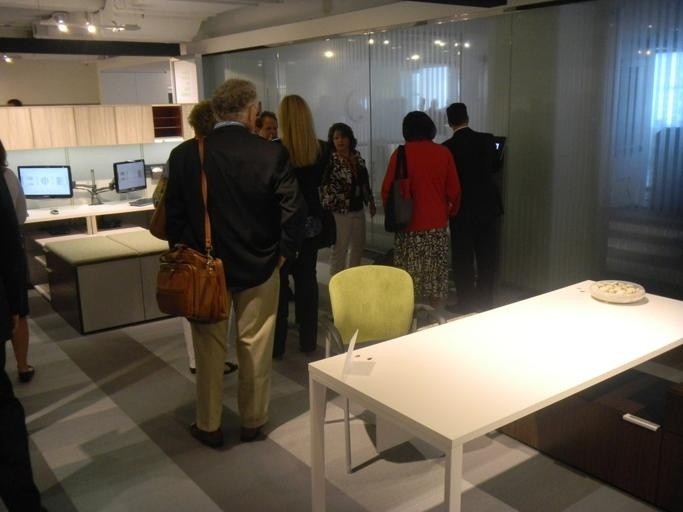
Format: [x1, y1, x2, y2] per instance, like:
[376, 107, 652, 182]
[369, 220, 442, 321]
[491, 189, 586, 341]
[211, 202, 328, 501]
[444, 293, 494, 315]
[189, 341, 316, 376]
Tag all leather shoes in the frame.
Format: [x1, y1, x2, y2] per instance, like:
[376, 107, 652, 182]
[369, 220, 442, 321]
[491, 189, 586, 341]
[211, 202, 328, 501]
[17, 363, 36, 382]
[239, 421, 262, 443]
[190, 419, 223, 451]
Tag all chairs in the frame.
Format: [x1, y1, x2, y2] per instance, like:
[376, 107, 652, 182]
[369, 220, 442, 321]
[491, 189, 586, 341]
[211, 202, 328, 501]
[316, 264, 450, 482]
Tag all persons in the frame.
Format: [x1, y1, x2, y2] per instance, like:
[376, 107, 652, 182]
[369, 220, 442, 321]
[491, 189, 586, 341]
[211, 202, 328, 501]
[0, 169, 51, 512]
[163, 74, 312, 450]
[148, 99, 240, 376]
[380, 107, 461, 325]
[253, 109, 276, 142]
[0, 141, 35, 386]
[440, 102, 505, 314]
[315, 119, 377, 323]
[266, 91, 330, 362]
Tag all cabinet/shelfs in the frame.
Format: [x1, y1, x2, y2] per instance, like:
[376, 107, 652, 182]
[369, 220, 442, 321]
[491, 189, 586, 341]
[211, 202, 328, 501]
[0, 103, 203, 149]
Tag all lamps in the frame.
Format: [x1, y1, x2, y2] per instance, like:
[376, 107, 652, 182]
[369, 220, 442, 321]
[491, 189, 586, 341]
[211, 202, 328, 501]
[38, 11, 137, 38]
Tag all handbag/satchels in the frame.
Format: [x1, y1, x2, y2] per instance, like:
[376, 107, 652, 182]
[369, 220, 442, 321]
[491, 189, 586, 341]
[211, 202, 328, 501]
[155, 247, 230, 326]
[356, 159, 370, 207]
[302, 205, 336, 251]
[383, 145, 413, 233]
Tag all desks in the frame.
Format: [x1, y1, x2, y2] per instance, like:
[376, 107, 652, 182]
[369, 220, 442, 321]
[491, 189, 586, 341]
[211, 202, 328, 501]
[18, 204, 144, 333]
[305, 276, 682, 510]
[91, 199, 175, 321]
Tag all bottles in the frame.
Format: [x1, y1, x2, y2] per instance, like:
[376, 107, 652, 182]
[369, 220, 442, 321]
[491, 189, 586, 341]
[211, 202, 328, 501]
[168, 86, 173, 105]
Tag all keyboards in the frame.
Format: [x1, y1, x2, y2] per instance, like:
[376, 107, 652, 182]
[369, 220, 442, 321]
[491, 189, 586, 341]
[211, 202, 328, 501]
[130, 197, 154, 207]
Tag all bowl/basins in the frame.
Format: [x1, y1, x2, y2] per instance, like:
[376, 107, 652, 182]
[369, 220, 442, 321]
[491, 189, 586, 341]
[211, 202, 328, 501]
[589, 279, 647, 305]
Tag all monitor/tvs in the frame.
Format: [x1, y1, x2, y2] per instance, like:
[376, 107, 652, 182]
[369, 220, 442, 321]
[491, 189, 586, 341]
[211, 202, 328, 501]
[113, 159, 146, 193]
[17, 165, 73, 198]
[493, 136, 506, 151]
